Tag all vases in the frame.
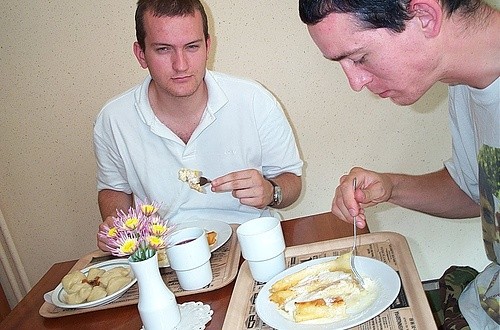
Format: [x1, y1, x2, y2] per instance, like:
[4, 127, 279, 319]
[128, 250, 181, 330]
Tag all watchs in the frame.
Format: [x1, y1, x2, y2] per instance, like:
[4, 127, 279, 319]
[264, 179, 283, 207]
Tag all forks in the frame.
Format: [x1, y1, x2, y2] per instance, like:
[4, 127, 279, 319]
[348, 177, 369, 290]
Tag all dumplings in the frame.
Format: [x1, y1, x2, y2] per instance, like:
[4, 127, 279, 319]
[61, 266, 131, 305]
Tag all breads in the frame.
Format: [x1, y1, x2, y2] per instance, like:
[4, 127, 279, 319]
[158, 231, 217, 260]
[269, 252, 353, 321]
[178, 168, 205, 192]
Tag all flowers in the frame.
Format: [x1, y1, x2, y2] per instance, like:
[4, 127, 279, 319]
[97, 196, 177, 261]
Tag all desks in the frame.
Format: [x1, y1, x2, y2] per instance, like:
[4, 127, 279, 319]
[0, 210, 370, 330]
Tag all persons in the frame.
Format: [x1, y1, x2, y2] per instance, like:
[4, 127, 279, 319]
[93, 0, 304, 254]
[299, 0, 500, 330]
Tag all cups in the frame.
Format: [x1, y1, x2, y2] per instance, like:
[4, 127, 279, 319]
[236, 217, 285, 282]
[165, 227, 213, 291]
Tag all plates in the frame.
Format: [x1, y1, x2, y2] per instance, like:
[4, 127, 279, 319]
[51, 259, 138, 308]
[254, 256, 401, 330]
[156, 220, 233, 268]
